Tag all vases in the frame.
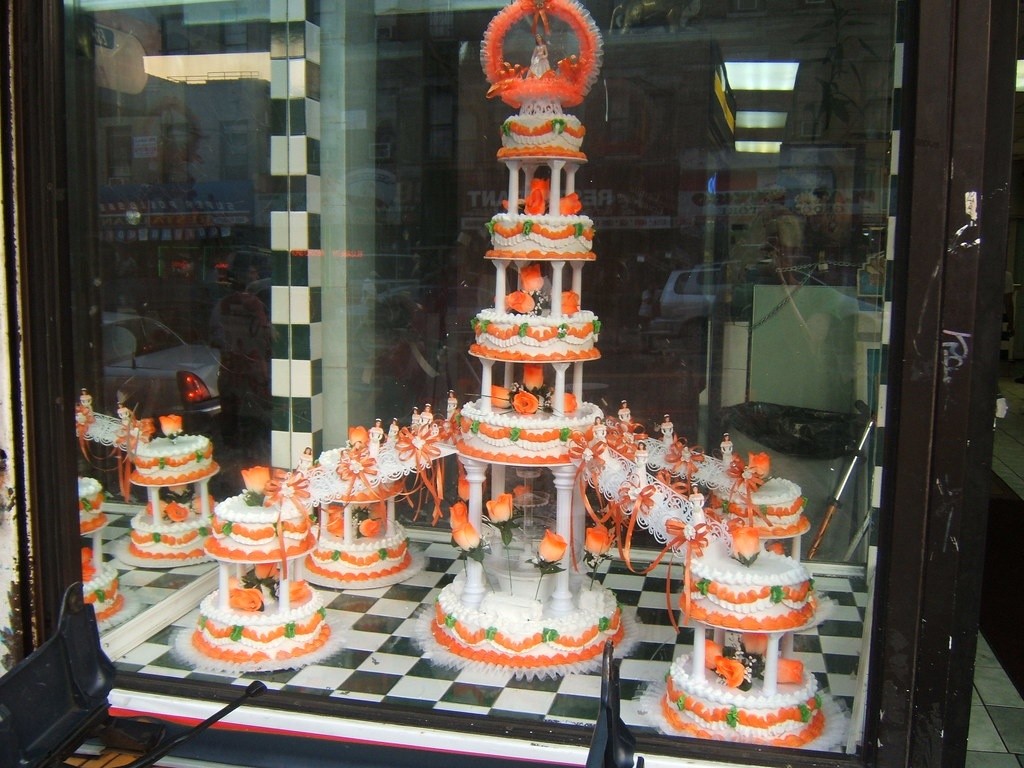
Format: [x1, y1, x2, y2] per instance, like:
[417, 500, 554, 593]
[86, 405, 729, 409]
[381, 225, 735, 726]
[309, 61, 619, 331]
[777, 214, 806, 251]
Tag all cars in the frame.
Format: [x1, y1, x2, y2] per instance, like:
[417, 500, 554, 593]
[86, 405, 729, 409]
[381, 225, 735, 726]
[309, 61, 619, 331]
[652, 242, 781, 371]
[98, 308, 222, 422]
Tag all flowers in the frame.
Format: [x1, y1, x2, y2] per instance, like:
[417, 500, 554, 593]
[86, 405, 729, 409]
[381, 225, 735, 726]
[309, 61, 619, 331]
[228, 562, 312, 612]
[762, 173, 825, 219]
[582, 524, 617, 590]
[148, 484, 214, 524]
[449, 502, 469, 576]
[465, 364, 579, 417]
[746, 450, 776, 492]
[486, 493, 522, 596]
[81, 546, 96, 581]
[240, 466, 271, 508]
[501, 177, 582, 216]
[526, 529, 568, 600]
[454, 523, 496, 594]
[730, 527, 763, 566]
[326, 505, 382, 539]
[493, 262, 580, 316]
[704, 631, 803, 691]
[160, 414, 183, 445]
[765, 541, 786, 558]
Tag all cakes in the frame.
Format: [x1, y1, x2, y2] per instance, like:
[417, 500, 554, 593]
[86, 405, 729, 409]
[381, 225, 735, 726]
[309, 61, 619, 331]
[73, 0, 828, 748]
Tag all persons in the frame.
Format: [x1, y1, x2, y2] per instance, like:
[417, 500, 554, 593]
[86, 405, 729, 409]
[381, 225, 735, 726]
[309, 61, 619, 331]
[79, 388, 93, 414]
[294, 447, 313, 480]
[634, 442, 649, 488]
[688, 484, 706, 533]
[411, 406, 421, 436]
[446, 390, 458, 420]
[210, 263, 273, 445]
[720, 432, 734, 468]
[592, 417, 608, 443]
[368, 417, 384, 456]
[518, 33, 566, 115]
[420, 403, 434, 437]
[639, 278, 663, 354]
[661, 414, 674, 446]
[117, 401, 131, 426]
[387, 418, 399, 448]
[618, 400, 631, 423]
